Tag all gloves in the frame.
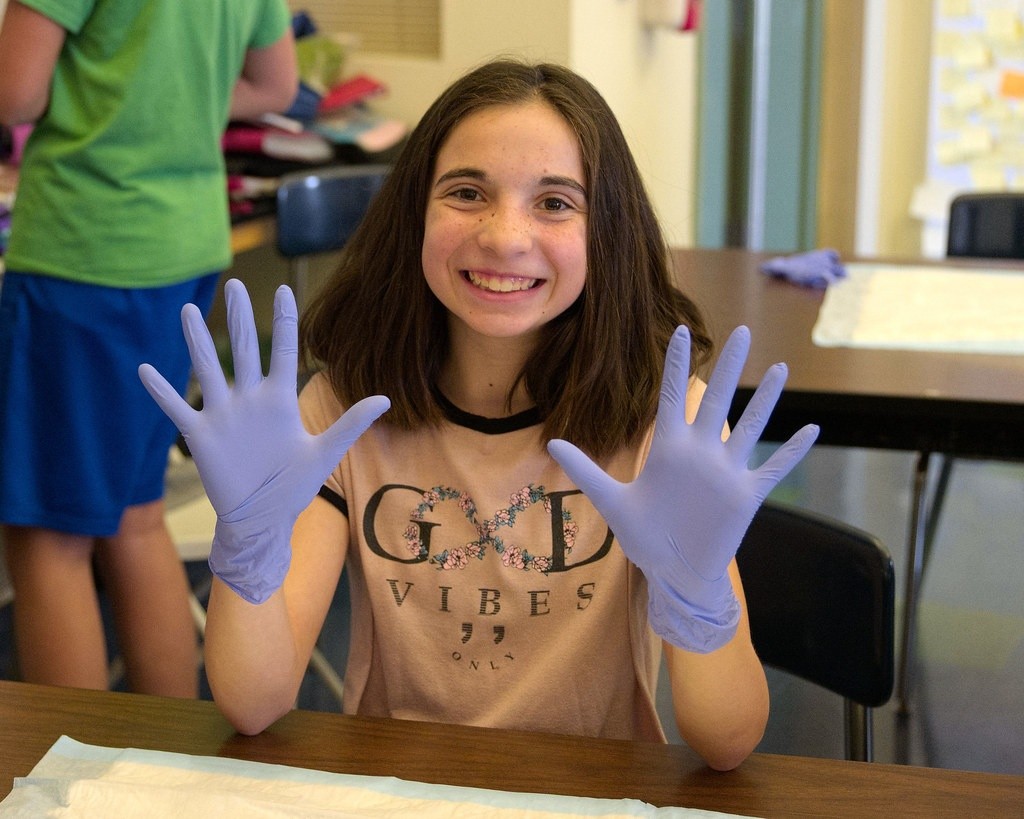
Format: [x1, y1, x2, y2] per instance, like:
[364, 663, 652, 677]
[545, 321, 823, 656]
[136, 276, 392, 608]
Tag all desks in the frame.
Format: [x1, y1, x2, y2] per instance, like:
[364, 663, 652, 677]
[672, 248, 1024, 466]
[0, 680, 1024, 819]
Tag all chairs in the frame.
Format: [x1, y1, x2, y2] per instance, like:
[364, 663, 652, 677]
[102, 442, 345, 709]
[277, 163, 391, 306]
[735, 500, 896, 762]
[893, 190, 1023, 719]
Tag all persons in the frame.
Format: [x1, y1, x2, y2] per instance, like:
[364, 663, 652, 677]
[137, 59, 820, 772]
[0, 0, 297, 698]
